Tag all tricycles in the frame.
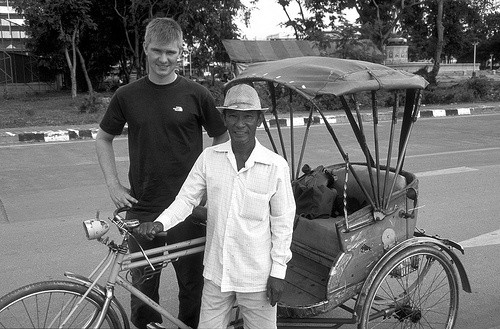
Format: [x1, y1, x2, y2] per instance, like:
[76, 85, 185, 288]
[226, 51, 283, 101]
[0, 56, 472, 329]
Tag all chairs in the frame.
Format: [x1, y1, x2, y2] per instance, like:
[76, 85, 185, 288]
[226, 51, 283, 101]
[290, 165, 406, 268]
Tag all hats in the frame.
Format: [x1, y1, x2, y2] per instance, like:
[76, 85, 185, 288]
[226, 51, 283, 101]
[215, 84, 268, 114]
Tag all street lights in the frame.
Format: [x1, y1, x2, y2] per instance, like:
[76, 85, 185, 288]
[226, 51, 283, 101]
[188, 46, 194, 79]
[471, 41, 479, 77]
[490, 54, 494, 74]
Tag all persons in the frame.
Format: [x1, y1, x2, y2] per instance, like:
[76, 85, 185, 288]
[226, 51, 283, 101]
[175, 63, 237, 87]
[94, 16, 233, 329]
[137, 83, 297, 329]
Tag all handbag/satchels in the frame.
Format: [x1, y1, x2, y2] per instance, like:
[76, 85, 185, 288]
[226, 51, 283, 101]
[291, 165, 337, 230]
